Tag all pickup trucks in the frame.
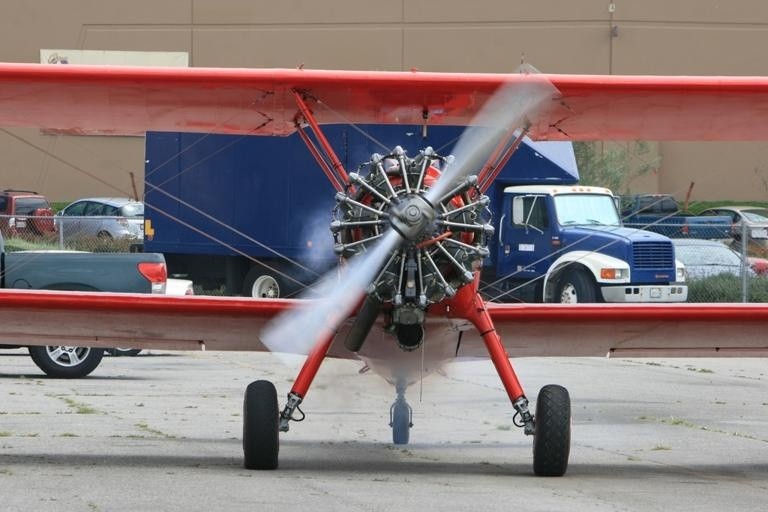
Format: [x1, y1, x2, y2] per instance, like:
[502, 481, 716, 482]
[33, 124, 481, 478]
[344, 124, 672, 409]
[0, 252, 168, 377]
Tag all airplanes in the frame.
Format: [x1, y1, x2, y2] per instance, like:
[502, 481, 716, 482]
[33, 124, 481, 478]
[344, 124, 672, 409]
[0, 62, 768, 477]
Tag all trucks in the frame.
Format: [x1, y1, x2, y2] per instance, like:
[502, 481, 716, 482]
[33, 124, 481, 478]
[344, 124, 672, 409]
[144, 124, 688, 302]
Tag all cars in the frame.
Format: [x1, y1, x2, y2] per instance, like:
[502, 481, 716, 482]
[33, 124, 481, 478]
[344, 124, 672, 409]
[623, 194, 767, 280]
[2, 189, 195, 357]
